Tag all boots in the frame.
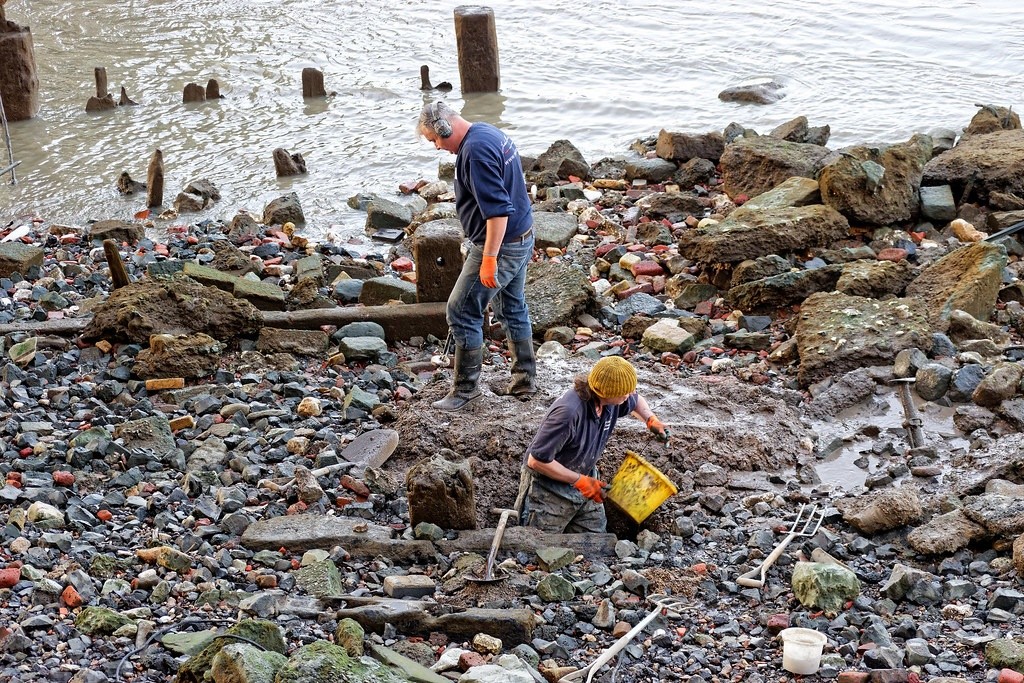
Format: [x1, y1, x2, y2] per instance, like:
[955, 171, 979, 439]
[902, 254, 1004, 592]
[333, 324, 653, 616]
[496, 334, 537, 401]
[431, 344, 483, 411]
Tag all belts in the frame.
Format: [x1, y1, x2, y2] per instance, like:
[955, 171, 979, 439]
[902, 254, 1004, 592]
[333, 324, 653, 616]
[502, 225, 534, 243]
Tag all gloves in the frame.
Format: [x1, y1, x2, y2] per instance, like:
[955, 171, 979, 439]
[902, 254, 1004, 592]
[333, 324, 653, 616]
[646, 413, 672, 447]
[571, 473, 612, 502]
[479, 252, 502, 288]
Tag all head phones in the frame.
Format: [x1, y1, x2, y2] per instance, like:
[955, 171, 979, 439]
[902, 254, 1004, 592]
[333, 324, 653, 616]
[431, 101, 453, 138]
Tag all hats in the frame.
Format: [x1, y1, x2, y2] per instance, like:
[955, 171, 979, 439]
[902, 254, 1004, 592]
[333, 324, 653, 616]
[587, 355, 636, 398]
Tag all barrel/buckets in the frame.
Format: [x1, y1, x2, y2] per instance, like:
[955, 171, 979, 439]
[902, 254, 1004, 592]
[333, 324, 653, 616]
[605, 438, 677, 525]
[776, 628, 827, 675]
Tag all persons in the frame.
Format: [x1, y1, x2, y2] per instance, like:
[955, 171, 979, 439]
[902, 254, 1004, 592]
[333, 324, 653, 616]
[519, 356, 671, 535]
[415, 101, 540, 411]
